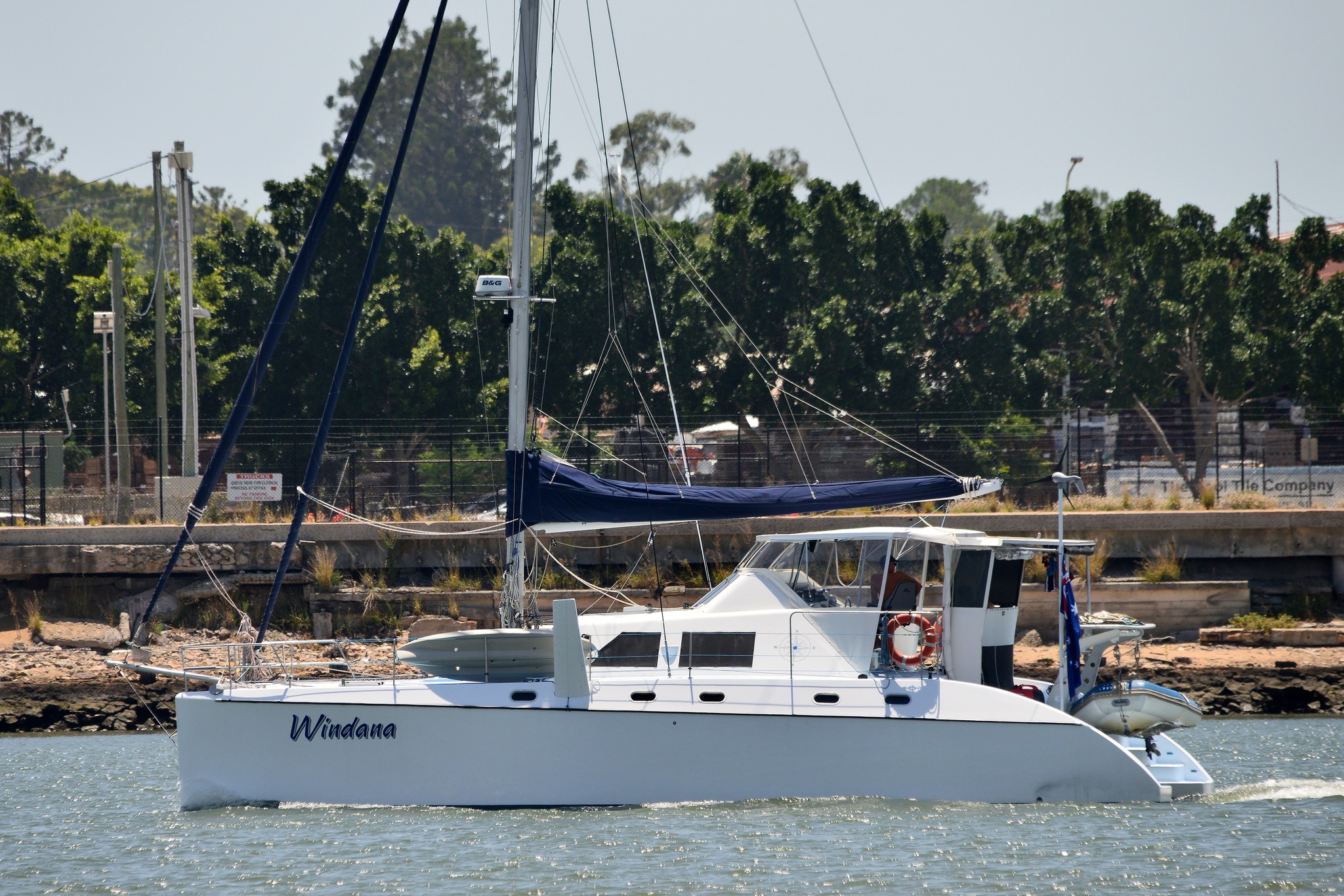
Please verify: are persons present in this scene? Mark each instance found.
[870,555,923,650]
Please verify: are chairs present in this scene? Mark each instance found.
[867,581,918,628]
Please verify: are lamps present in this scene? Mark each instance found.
[996,547,1034,561]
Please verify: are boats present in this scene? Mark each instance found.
[1064,675,1202,736]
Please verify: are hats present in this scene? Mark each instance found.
[879,554,899,566]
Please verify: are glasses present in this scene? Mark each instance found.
[889,562,897,566]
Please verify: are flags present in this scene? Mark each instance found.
[1060,559,1082,701]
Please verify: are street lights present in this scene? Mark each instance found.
[168,140,211,478]
[1066,156,1084,203]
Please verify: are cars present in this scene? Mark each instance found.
[464,488,506,514]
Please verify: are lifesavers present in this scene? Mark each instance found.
[933,616,943,654]
[886,613,936,666]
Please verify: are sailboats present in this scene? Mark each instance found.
[101,0,1218,812]
